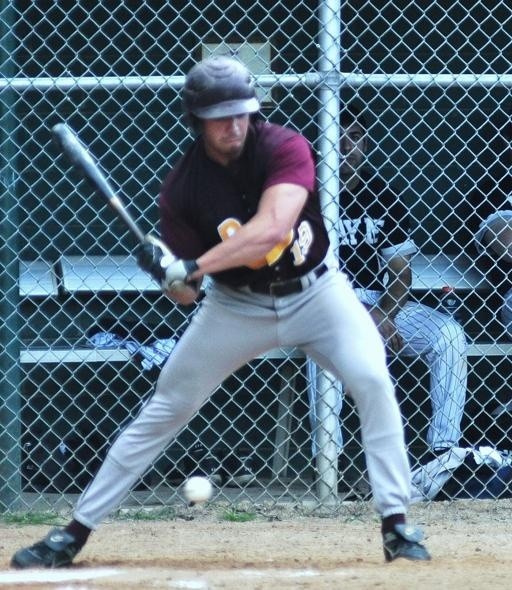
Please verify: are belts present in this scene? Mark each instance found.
[231,263,334,295]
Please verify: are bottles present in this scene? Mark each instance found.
[439,286,464,328]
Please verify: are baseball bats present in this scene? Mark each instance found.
[53,120,184,296]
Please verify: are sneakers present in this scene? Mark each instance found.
[9,527,76,569]
[378,524,432,565]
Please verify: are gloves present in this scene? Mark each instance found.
[163,257,197,288]
[136,235,173,275]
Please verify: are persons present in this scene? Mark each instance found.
[304,104,472,495]
[469,177,511,430]
[10,54,432,569]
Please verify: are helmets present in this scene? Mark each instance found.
[183,56,261,123]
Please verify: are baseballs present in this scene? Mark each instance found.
[183,476,212,500]
